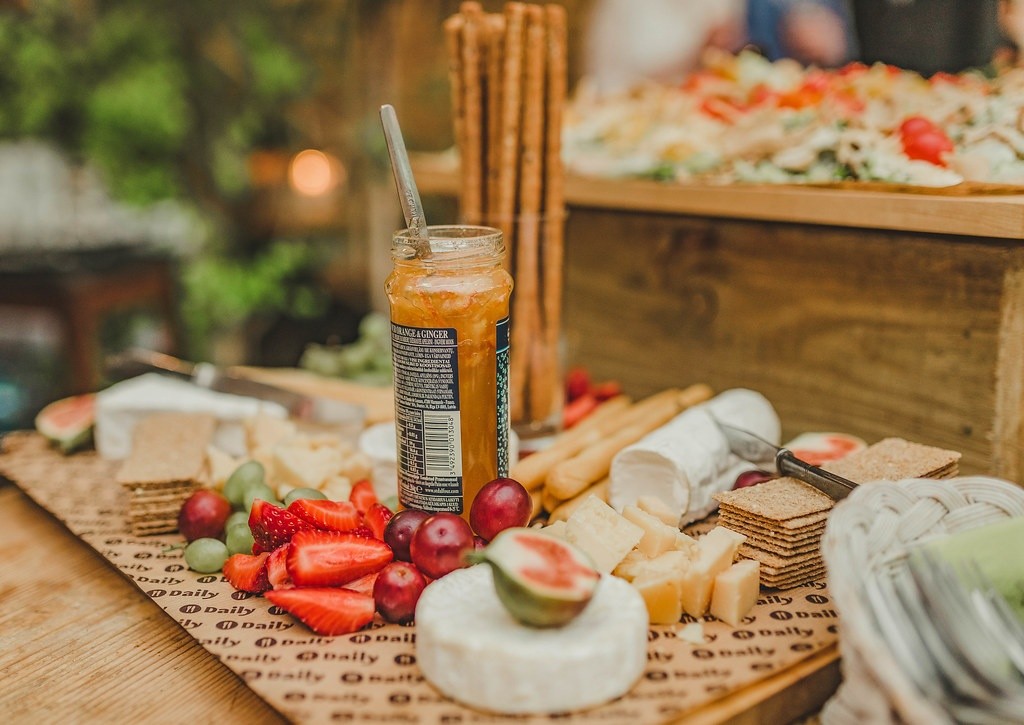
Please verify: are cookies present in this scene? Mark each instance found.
[709,437,963,590]
[118,414,216,533]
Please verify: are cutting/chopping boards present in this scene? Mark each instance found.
[667,644,845,725]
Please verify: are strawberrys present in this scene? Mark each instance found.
[224,478,395,635]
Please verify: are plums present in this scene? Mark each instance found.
[373,477,534,624]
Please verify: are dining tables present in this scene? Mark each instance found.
[0,366,953,725]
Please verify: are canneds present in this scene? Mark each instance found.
[384,225,514,514]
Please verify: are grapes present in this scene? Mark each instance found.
[183,460,328,576]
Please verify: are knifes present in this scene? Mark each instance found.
[718,420,860,501]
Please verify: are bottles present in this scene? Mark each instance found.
[384,225,514,544]
